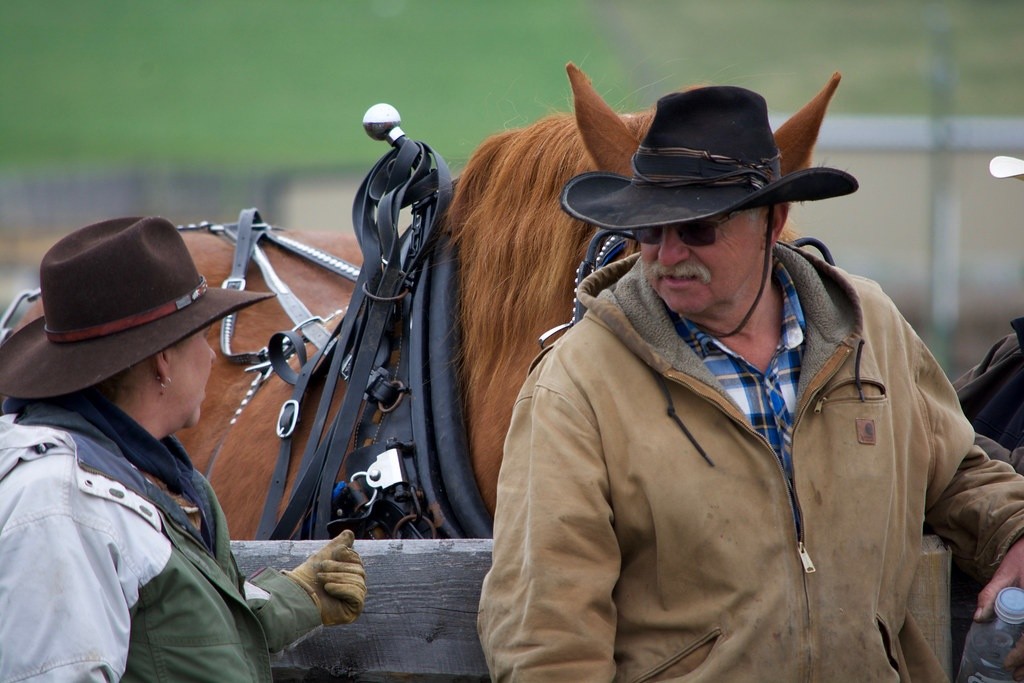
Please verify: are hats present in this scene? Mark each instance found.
[560,86,859,232]
[0,216,277,398]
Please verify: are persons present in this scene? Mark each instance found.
[0,215,368,683]
[477,84,1024,683]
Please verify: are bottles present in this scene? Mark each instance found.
[956,588,1024,683]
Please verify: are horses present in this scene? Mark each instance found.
[1,63,844,540]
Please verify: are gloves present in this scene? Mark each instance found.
[279,530,368,626]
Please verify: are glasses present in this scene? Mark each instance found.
[632,210,745,246]
